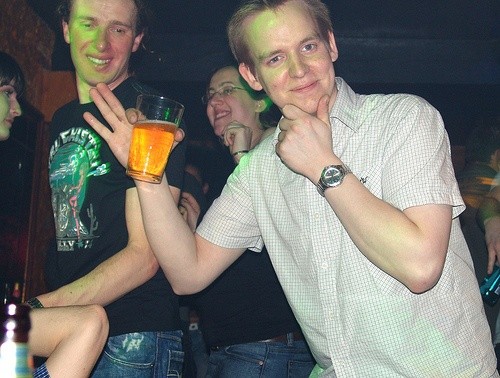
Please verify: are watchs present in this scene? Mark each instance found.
[317,164,352,197]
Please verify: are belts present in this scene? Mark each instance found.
[256,332,305,351]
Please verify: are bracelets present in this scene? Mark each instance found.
[233,150,249,156]
[26,297,44,308]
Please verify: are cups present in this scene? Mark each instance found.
[126,94,185,184]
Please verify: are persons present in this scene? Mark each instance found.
[25,0,187,378]
[179,63,317,378]
[457,125,500,337]
[83,0,500,378]
[0,51,23,138]
[26,303,110,378]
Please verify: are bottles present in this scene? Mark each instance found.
[479,265,500,308]
[0,283,34,378]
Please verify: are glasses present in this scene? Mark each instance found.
[200,86,252,105]
